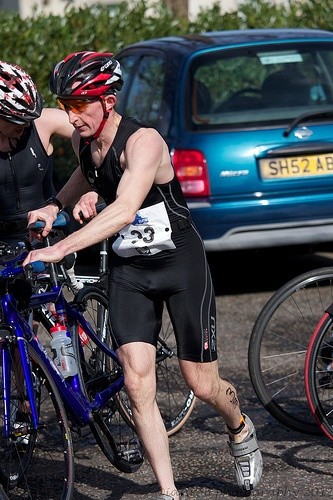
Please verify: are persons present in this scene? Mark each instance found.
[0,62,82,461]
[23,51,263,500]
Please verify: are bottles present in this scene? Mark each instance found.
[77,326,89,348]
[50,323,78,377]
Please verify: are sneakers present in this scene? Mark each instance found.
[226,413,264,492]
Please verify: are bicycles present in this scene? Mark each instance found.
[0,208,199,500]
[247,266,333,443]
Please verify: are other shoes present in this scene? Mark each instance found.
[7,421,37,448]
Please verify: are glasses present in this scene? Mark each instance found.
[54,96,100,115]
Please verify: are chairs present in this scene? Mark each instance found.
[260,71,311,109]
[194,80,212,116]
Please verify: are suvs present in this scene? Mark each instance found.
[112,25,333,272]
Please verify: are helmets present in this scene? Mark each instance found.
[48,50,125,98]
[0,60,43,124]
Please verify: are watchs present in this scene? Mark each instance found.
[46,196,63,213]
